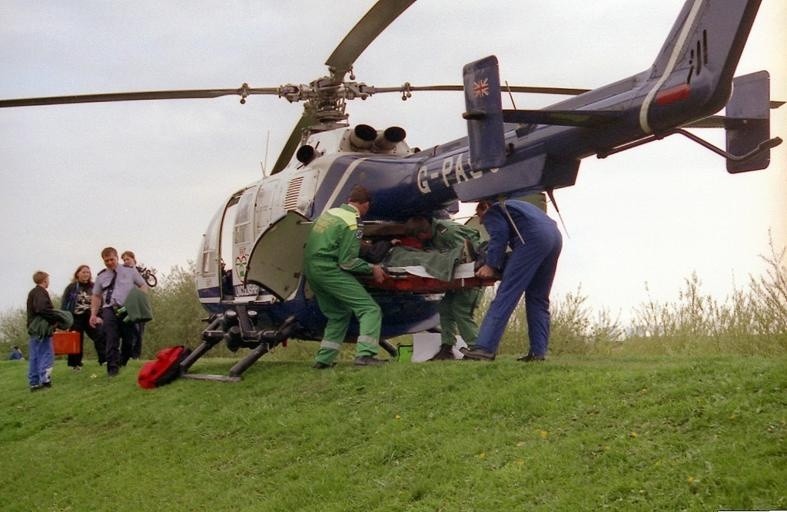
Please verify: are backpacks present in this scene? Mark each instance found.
[138,344,193,389]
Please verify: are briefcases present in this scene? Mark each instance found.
[52,330,83,355]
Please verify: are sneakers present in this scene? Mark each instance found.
[426,351,454,362]
[313,360,338,369]
[516,355,545,362]
[354,355,389,366]
[460,345,495,360]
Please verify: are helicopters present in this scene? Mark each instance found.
[1,0,787,386]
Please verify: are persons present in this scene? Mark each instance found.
[358,238,477,266]
[403,214,488,362]
[9,345,23,360]
[26,271,55,392]
[89,247,148,380]
[458,199,563,363]
[302,183,391,370]
[59,264,108,371]
[121,250,149,362]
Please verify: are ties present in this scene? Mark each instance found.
[101,270,117,304]
